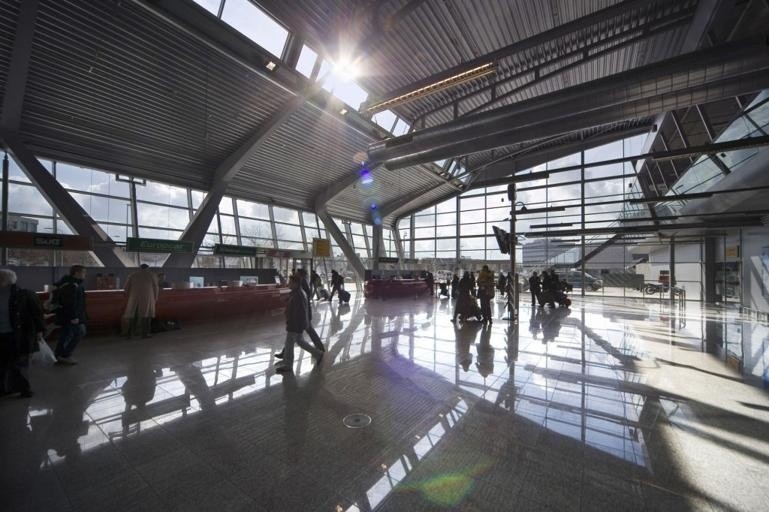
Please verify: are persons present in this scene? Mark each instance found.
[0,268,43,399]
[54,265,87,365]
[96,263,169,339]
[421,265,571,325]
[274,269,344,373]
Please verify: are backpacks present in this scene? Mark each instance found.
[39,283,64,315]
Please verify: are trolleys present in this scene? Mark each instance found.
[436,279,451,299]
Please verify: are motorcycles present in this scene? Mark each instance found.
[645,283,668,295]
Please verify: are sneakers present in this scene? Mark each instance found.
[450,317,493,325]
[7,388,38,403]
[126,332,139,341]
[311,343,328,375]
[274,346,292,360]
[54,355,80,368]
[530,304,556,311]
[274,364,294,375]
[141,331,155,342]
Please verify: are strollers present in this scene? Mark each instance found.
[458,289,483,324]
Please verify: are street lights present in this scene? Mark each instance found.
[403,234,407,253]
[43,226,62,234]
[123,203,133,242]
[503,201,528,320]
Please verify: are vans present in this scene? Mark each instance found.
[437,269,450,279]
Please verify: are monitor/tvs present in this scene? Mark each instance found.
[491,225,510,253]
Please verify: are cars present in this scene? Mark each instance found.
[560,269,604,291]
